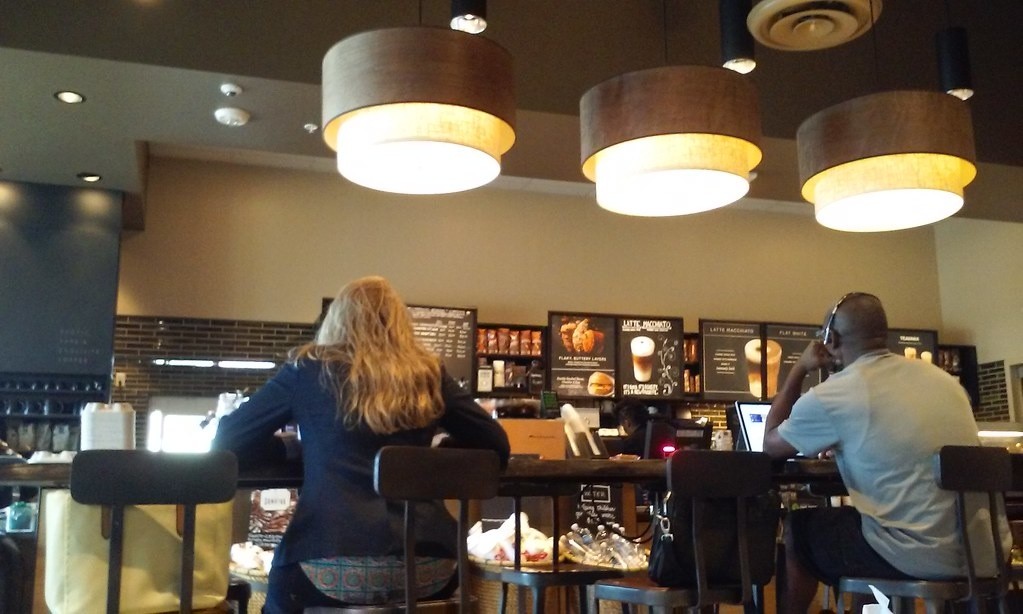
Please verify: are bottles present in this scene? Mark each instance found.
[560,521,646,567]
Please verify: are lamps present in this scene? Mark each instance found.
[578,0,764,218]
[935,0,975,101]
[449,0,488,33]
[718,0,756,74]
[794,0,977,233]
[321,0,517,193]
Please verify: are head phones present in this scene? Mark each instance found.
[824,292,879,345]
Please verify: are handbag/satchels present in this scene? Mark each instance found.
[646,491,696,588]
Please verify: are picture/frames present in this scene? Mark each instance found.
[887,329,938,368]
[404,304,477,397]
[617,313,685,401]
[547,309,617,401]
[764,321,828,404]
[698,317,764,405]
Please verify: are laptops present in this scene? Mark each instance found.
[734,400,807,459]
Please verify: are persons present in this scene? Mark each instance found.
[610,395,656,506]
[762,291,1014,614]
[210,276,512,614]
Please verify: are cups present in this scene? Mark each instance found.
[744,339,782,397]
[1,380,103,391]
[630,336,654,383]
[905,346,917,359]
[921,351,932,363]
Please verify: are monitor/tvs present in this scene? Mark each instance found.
[645,417,712,460]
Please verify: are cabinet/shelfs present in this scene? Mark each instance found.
[475,323,547,397]
[681,331,700,403]
[935,342,979,413]
[0,372,114,459]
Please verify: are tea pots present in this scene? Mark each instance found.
[1,396,93,415]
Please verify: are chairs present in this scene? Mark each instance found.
[0,446,1023,614]
